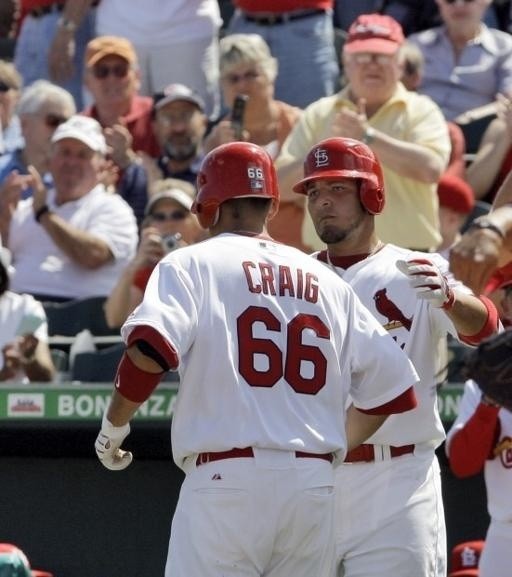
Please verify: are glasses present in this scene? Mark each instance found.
[0,83,10,91]
[151,210,187,221]
[357,54,391,65]
[94,65,127,78]
[46,115,66,127]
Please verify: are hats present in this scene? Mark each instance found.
[0,543,52,576]
[84,36,135,68]
[145,178,196,211]
[151,83,206,113]
[449,541,485,577]
[437,175,474,214]
[52,115,106,155]
[344,15,405,55]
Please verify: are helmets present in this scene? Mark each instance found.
[292,137,384,213]
[192,141,280,229]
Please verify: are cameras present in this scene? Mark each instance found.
[160,234,181,253]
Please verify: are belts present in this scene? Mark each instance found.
[196,446,334,463]
[344,445,414,462]
[244,8,325,26]
[31,3,65,18]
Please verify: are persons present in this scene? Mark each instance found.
[288,134,510,574]
[1,0,511,397]
[92,135,420,574]
[442,265,511,576]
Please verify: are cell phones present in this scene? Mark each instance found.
[229,94,245,138]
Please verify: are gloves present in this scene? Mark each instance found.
[94,410,133,470]
[397,258,454,310]
